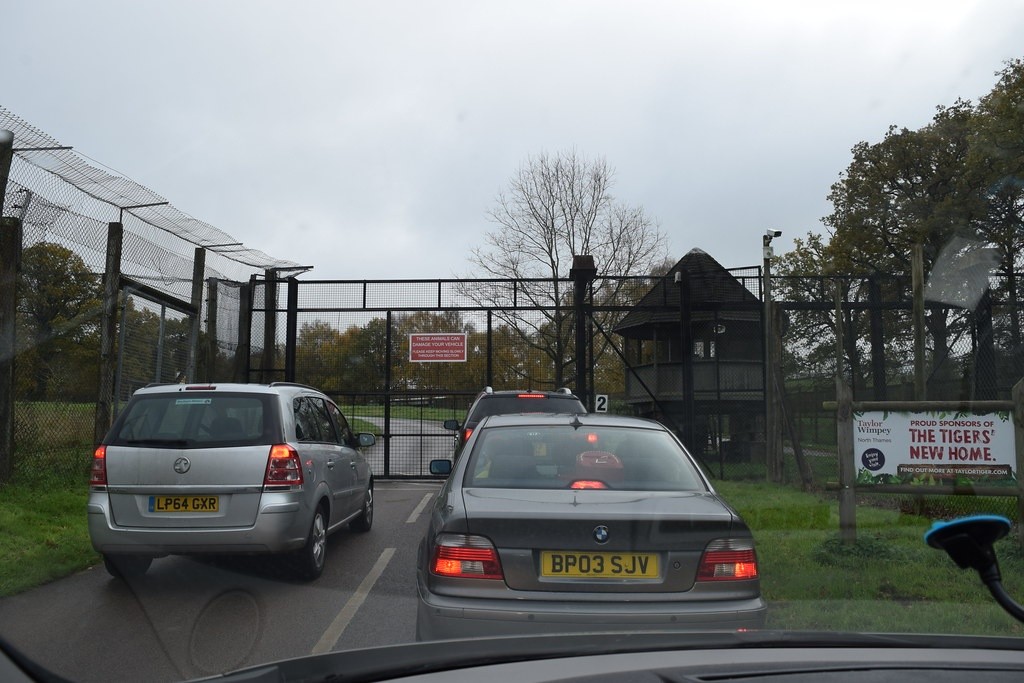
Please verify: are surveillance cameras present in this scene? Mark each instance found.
[767,229,782,237]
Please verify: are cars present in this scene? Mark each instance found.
[87,379,376,583]
[416,412,767,643]
[444,386,587,463]
